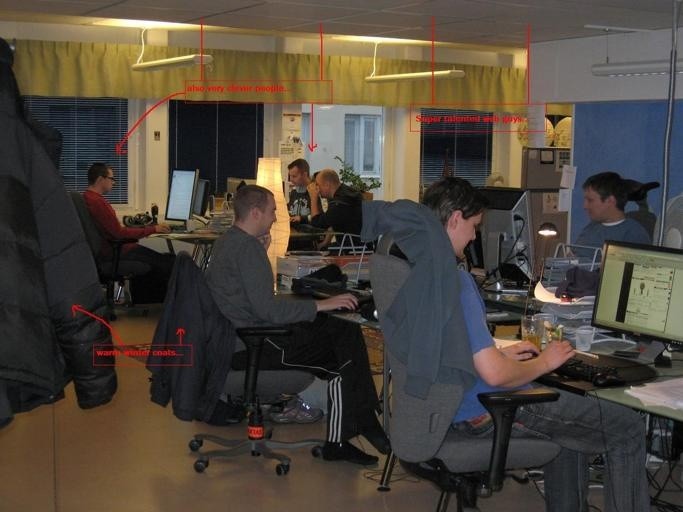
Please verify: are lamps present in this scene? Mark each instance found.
[365,43,465,84]
[591,26,683,78]
[132,29,212,72]
[537,224,559,238]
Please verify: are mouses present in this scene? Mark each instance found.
[338,301,355,315]
[593,371,626,388]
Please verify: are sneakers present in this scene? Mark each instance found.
[268,395,324,424]
[363,424,392,455]
[322,440,379,465]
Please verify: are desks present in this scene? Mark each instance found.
[150,213,328,256]
[281,274,683,422]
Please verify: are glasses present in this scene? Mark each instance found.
[102,175,115,182]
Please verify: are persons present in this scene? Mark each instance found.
[305,171,362,253]
[84,162,175,285]
[200,185,388,464]
[287,159,318,224]
[420,179,651,511]
[565,173,650,263]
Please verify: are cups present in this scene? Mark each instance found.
[151,205,158,224]
[521,313,595,353]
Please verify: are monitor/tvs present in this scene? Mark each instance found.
[464,187,537,292]
[591,238,683,366]
[165,166,199,224]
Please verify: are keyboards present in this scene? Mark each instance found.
[554,357,621,382]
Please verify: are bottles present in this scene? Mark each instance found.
[295,266,304,279]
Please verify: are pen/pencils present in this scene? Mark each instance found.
[573,349,599,360]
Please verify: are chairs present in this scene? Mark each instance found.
[325,232,370,255]
[367,252,563,510]
[67,189,149,321]
[627,211,659,242]
[176,249,329,476]
